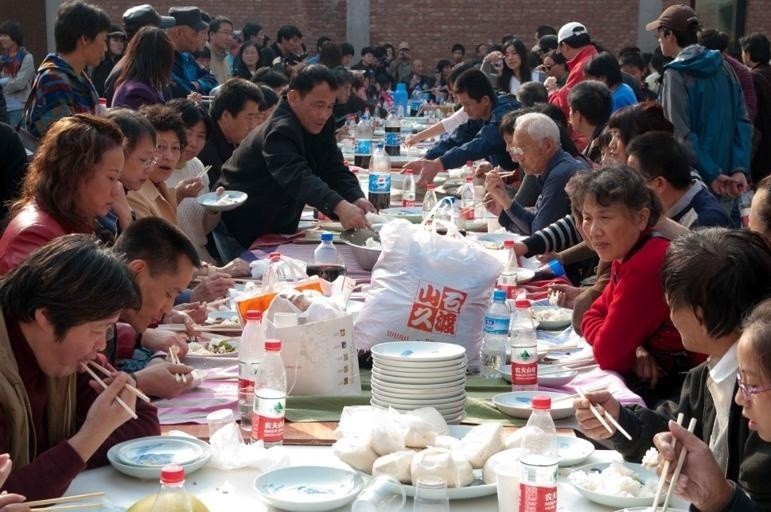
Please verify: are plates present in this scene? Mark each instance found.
[173,309,245,358]
[476,233,581,420]
[332,424,500,501]
[295,206,348,233]
[513,435,592,465]
[569,459,677,506]
[253,463,362,510]
[105,435,212,478]
[196,188,249,212]
[371,341,470,425]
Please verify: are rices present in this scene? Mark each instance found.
[569,446,666,498]
[362,237,381,250]
[535,295,569,321]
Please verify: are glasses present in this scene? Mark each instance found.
[510,142,535,156]
[736,370,771,400]
[143,157,159,169]
[542,63,557,71]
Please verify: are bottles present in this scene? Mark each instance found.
[97,97,110,116]
[312,232,348,279]
[236,310,290,449]
[148,463,200,510]
[518,393,559,510]
[262,252,295,284]
[347,85,476,227]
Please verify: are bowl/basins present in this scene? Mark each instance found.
[340,223,383,271]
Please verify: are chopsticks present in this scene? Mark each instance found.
[167,343,188,387]
[187,94,216,103]
[461,196,495,216]
[76,358,151,421]
[9,490,111,510]
[485,171,515,180]
[577,389,633,441]
[192,164,212,184]
[539,341,609,401]
[648,412,698,510]
[155,272,264,333]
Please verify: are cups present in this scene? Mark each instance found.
[206,409,239,466]
[349,477,452,509]
[494,460,520,510]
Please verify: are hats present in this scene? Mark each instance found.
[169,7,209,30]
[646,5,699,32]
[557,22,588,54]
[201,9,217,27]
[398,41,410,51]
[107,25,127,38]
[122,4,176,31]
[532,35,558,52]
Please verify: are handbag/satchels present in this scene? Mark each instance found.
[256,311,361,398]
[14,125,42,163]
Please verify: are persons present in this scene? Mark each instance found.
[1,1,771,512]
